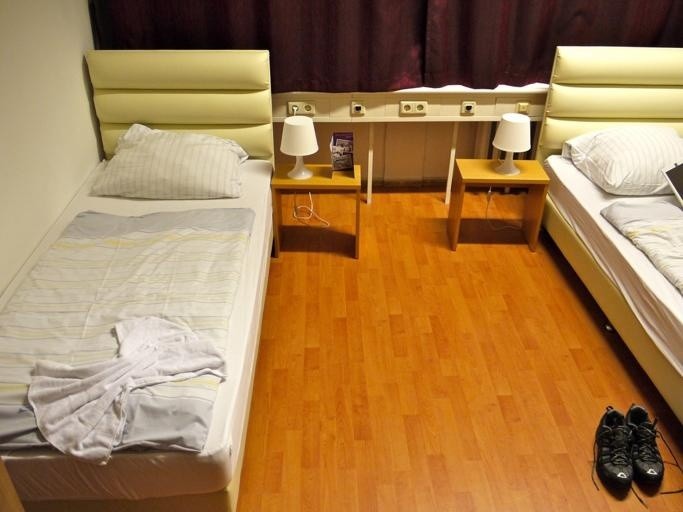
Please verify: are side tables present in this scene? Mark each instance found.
[271,164,362,258]
[448,158,550,251]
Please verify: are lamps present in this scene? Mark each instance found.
[492,113,532,176]
[280,115,319,180]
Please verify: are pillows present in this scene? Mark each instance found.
[562,127,683,196]
[91,123,248,200]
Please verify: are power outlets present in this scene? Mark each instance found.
[399,100,428,114]
[288,102,315,115]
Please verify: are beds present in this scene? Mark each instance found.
[535,45,683,425]
[0,49,274,512]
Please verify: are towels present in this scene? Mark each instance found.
[26,317,227,465]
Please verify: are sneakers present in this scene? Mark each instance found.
[591,403,664,490]
